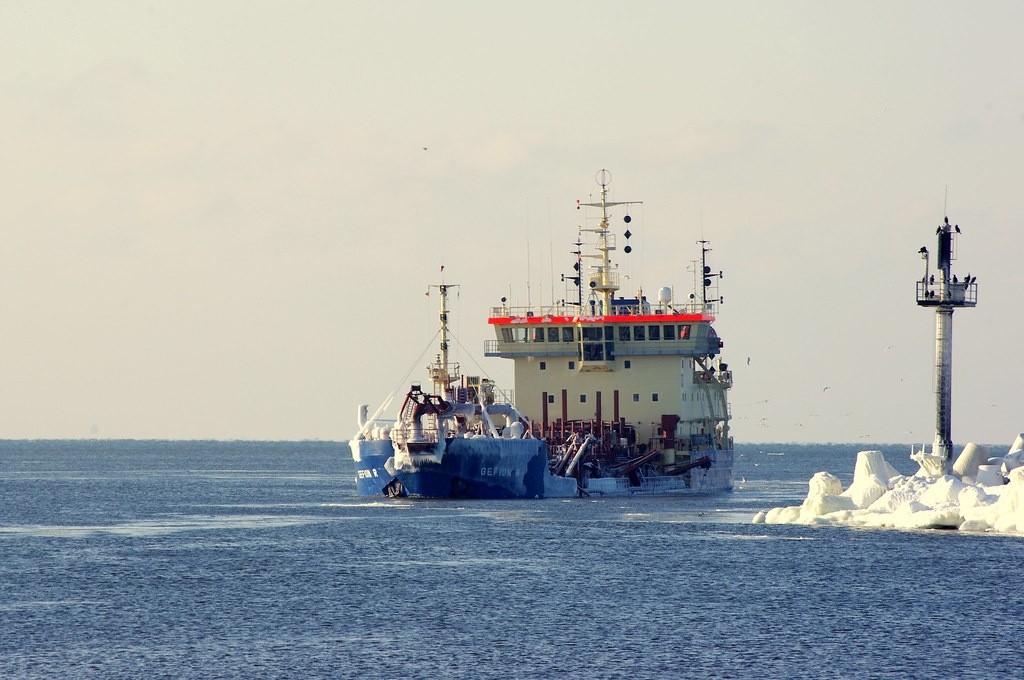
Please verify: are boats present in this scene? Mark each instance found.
[481,169,734,500]
[348,263,551,501]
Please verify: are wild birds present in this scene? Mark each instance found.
[918,246,928,254]
[952,273,976,290]
[936,215,963,235]
[741,475,746,484]
[822,386,831,393]
[746,356,751,367]
[922,274,935,299]
